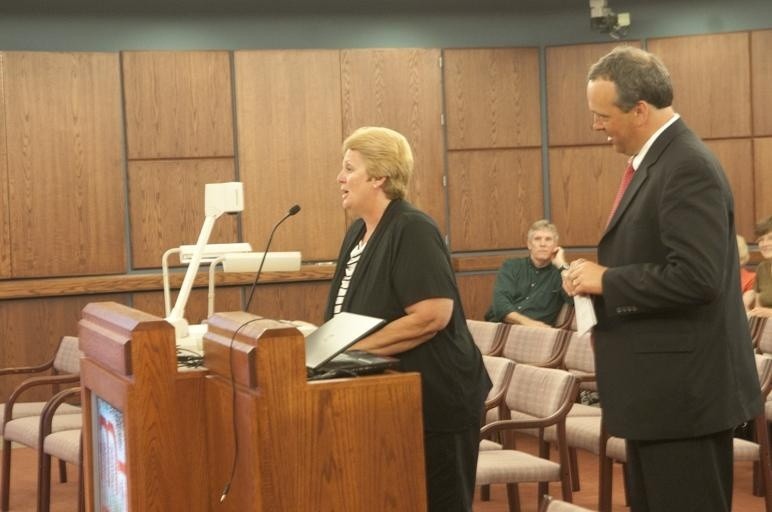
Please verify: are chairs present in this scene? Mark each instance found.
[1,302,772,512]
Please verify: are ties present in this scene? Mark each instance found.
[602,158,637,239]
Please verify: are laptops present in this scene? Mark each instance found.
[304,311,401,377]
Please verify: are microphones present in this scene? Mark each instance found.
[244,205,302,314]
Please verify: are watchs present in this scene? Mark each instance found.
[556,262,570,274]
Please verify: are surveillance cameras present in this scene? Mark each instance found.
[589,0,618,32]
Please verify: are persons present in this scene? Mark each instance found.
[323,126,494,512]
[735,233,755,311]
[559,43,764,511]
[743,216,771,320]
[483,216,574,329]
